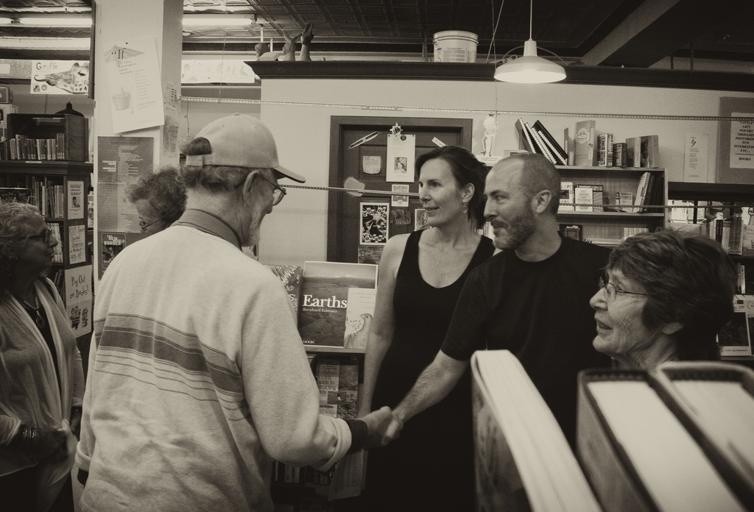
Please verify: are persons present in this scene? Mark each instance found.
[358,143,500,511]
[0,201,86,511]
[72,111,394,511]
[376,149,614,447]
[588,228,729,369]
[121,164,184,242]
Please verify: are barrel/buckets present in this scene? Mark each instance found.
[431,29,481,63]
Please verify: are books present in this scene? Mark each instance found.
[570,361,744,511]
[511,115,753,267]
[255,262,375,492]
[639,353,752,509]
[9,109,69,298]
[457,348,602,509]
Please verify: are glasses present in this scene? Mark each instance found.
[20,228,54,243]
[262,172,287,206]
[598,273,649,302]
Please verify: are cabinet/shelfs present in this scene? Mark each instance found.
[666,181,754,366]
[550,165,665,253]
[260,265,380,512]
[0,159,94,390]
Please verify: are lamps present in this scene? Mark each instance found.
[492,0,566,85]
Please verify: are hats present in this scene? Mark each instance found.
[181,114,308,183]
